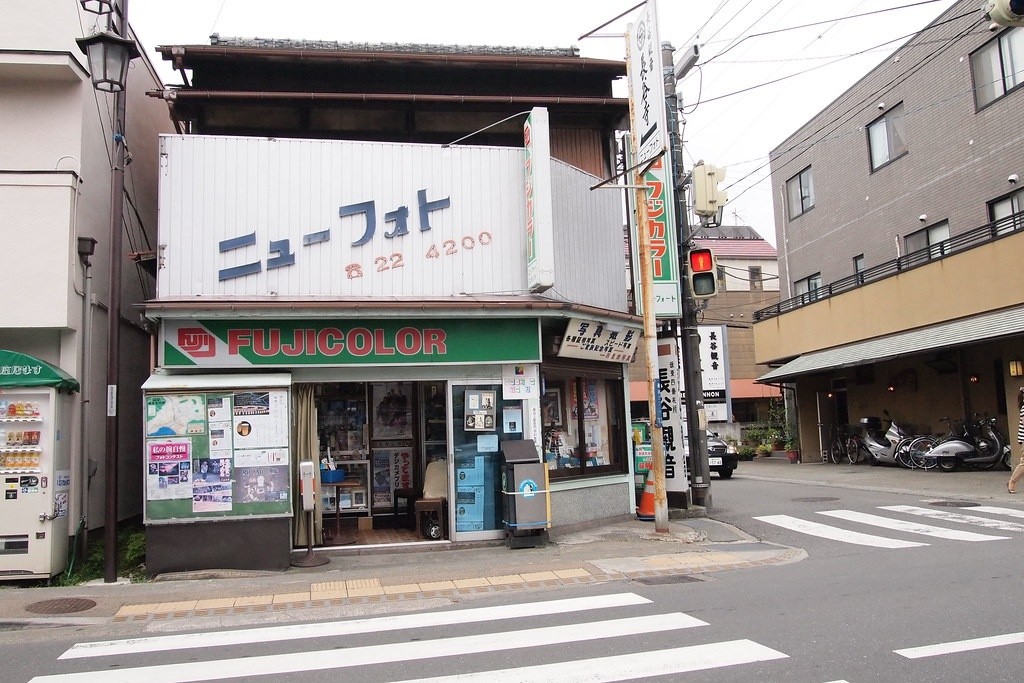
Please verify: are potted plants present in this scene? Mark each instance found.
[784,441,798,463]
[738,424,786,461]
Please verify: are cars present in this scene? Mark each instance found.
[680,417,740,479]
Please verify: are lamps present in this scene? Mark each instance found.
[1009,358,1023,377]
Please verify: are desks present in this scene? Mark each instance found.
[320,478,363,545]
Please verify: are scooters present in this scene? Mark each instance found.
[923,409,1011,472]
[858,410,922,469]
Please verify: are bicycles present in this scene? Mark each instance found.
[895,412,975,470]
[829,422,861,465]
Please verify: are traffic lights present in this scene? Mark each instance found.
[689,247,720,300]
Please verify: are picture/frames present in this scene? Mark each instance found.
[367,382,419,448]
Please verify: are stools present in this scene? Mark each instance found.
[415,498,446,540]
[393,487,421,531]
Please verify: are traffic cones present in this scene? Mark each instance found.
[636,462,656,521]
